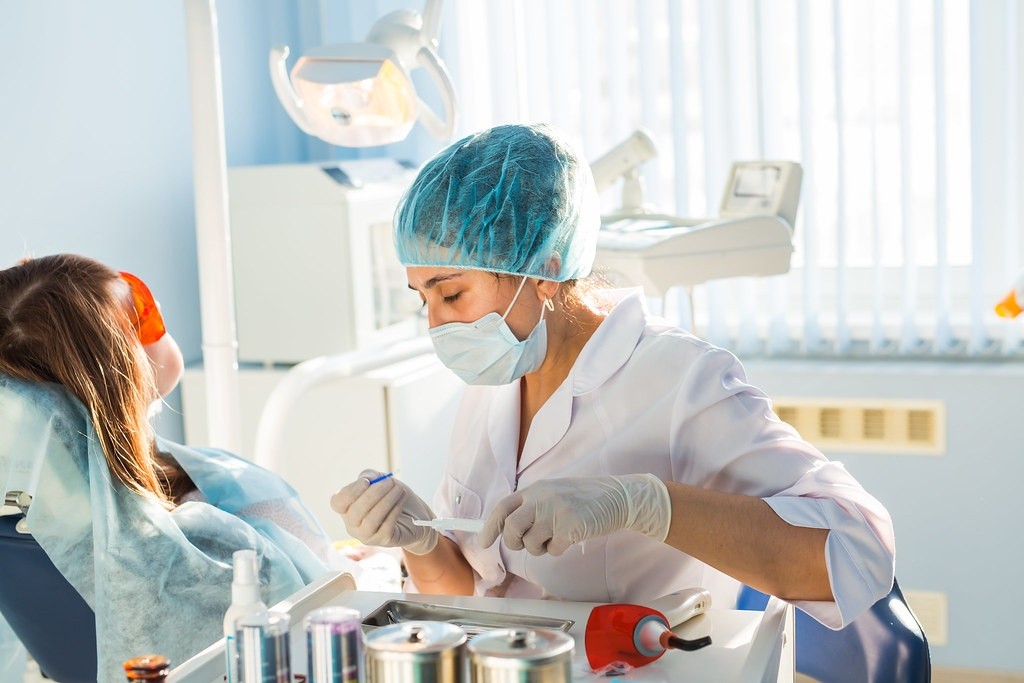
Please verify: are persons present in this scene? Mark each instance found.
[330,125,895,683]
[0,252,402,595]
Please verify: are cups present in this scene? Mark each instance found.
[362,619,468,683]
[468,625,577,683]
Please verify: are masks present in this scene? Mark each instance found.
[428,261,550,385]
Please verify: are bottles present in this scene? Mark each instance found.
[124,653,171,683]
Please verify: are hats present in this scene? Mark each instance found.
[392,123,600,281]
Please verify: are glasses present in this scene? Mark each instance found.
[122,271,167,349]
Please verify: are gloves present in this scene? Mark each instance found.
[477,472,672,554]
[331,470,440,553]
